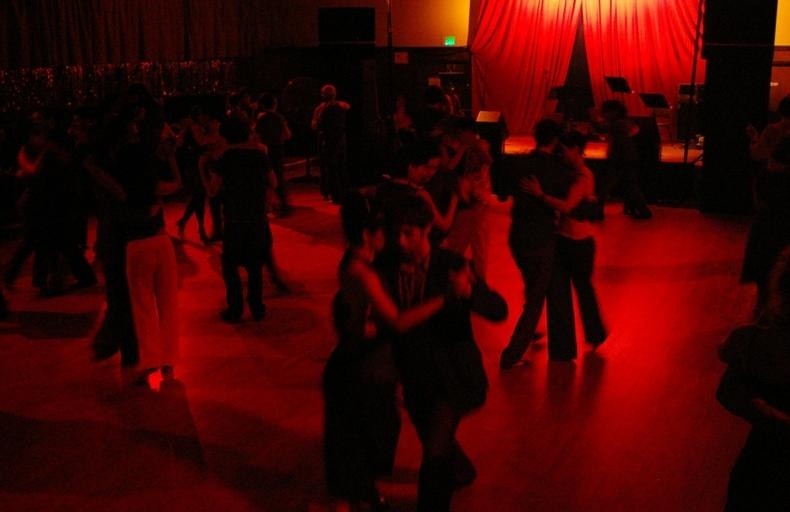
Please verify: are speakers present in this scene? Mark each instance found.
[319,7,375,42]
[477,110,509,139]
[700,48,772,216]
[704,0,777,47]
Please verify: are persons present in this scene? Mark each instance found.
[2,83,787,509]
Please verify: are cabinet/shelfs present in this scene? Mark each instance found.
[357,43,470,128]
